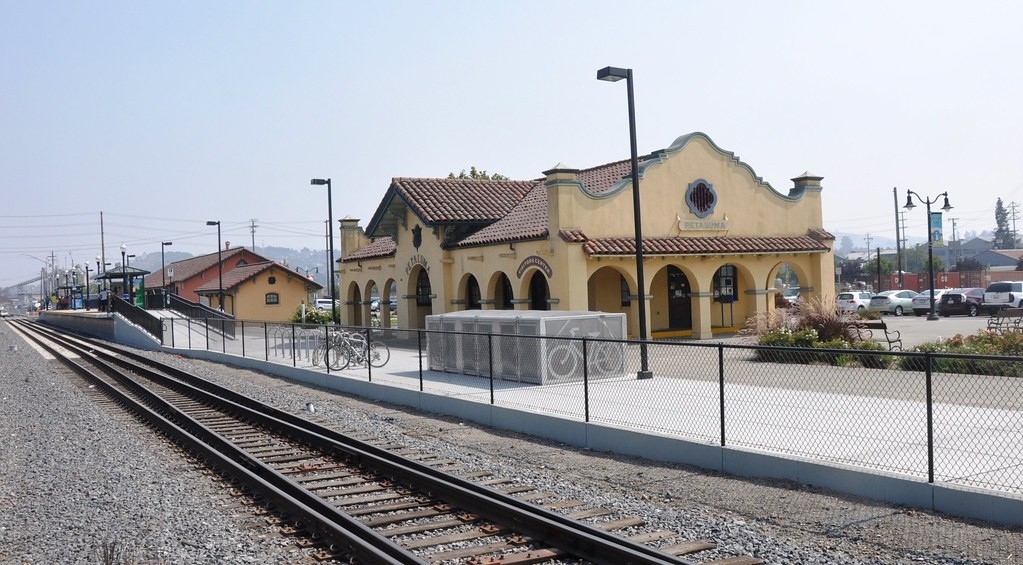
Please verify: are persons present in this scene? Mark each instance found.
[56,295,70,310]
[51,293,56,310]
[45,296,50,310]
[99,289,107,312]
[114,286,123,296]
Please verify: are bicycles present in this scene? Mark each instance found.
[312,326,390,372]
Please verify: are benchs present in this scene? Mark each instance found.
[845,322,903,352]
[986,304,1023,336]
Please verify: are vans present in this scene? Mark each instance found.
[981,281,1023,310]
[313,299,333,310]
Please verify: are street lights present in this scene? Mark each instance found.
[55,261,90,311]
[597,66,653,379]
[96,254,101,311]
[309,178,337,325]
[902,189,954,482]
[120,241,127,294]
[126,255,135,267]
[206,221,223,311]
[161,241,173,289]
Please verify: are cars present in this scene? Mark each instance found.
[782,287,802,304]
[836,286,986,317]
[390,300,397,311]
